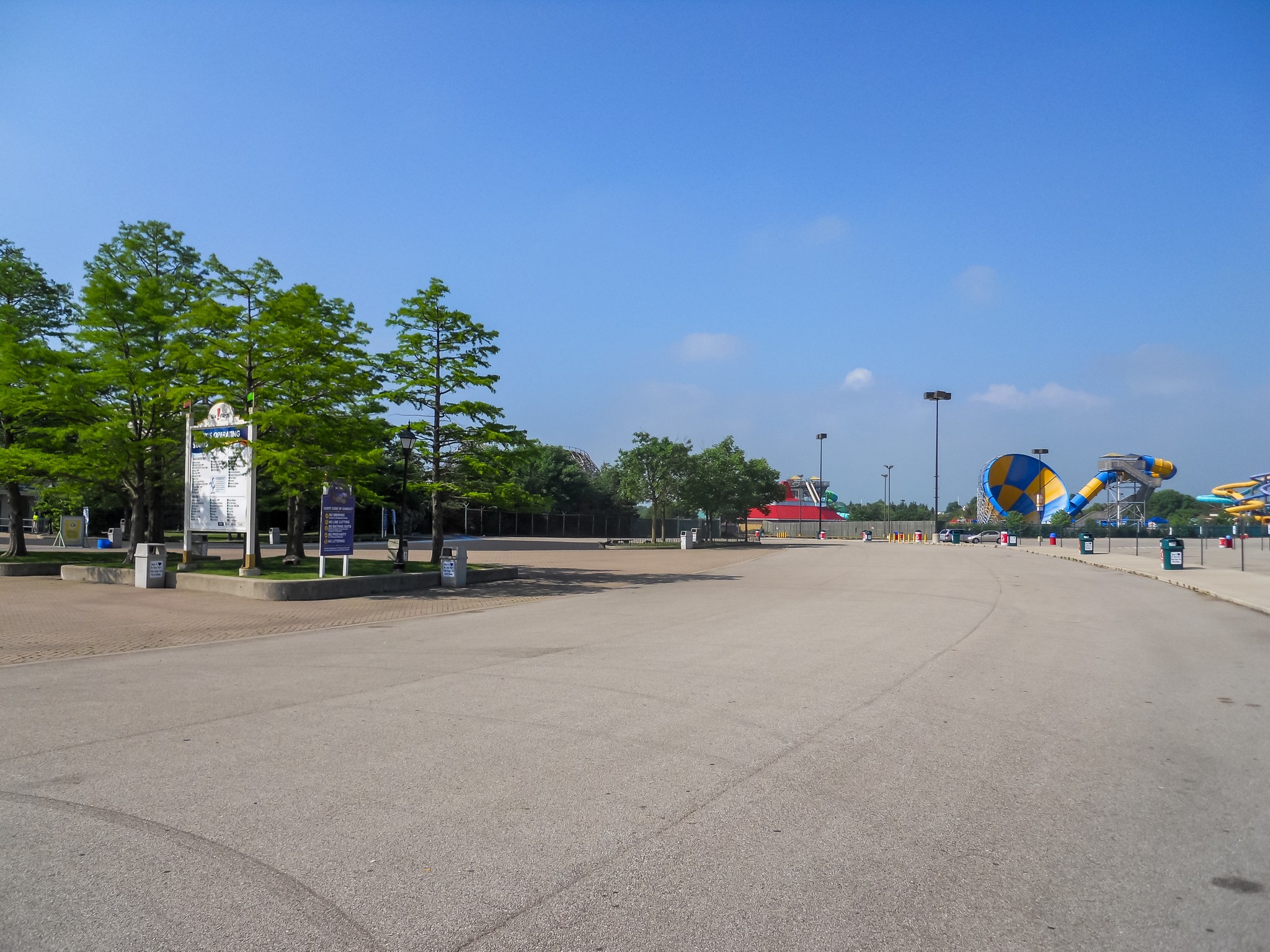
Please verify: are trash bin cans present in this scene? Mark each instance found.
[191,534,209,556]
[1079,531,1094,554]
[680,530,693,549]
[915,529,922,542]
[134,543,168,588]
[268,528,280,545]
[899,532,903,540]
[755,530,764,538]
[107,528,122,548]
[387,539,409,561]
[817,529,826,540]
[894,531,898,540]
[1001,530,1018,546]
[1159,536,1185,570]
[951,531,961,544]
[439,546,468,587]
[1219,535,1232,549]
[862,530,872,541]
[690,528,702,543]
[1049,533,1056,545]
[120,519,131,541]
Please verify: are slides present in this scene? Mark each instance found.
[1196,472,1270,526]
[822,491,838,504]
[977,453,1176,525]
[836,512,850,518]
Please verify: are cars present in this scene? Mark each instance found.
[967,530,1000,544]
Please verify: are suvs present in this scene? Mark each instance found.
[939,528,977,543]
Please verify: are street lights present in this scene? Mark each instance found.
[393,420,418,572]
[797,475,804,536]
[924,390,952,543]
[46,483,55,535]
[884,464,893,539]
[1031,449,1049,547]
[816,433,827,533]
[881,474,887,537]
